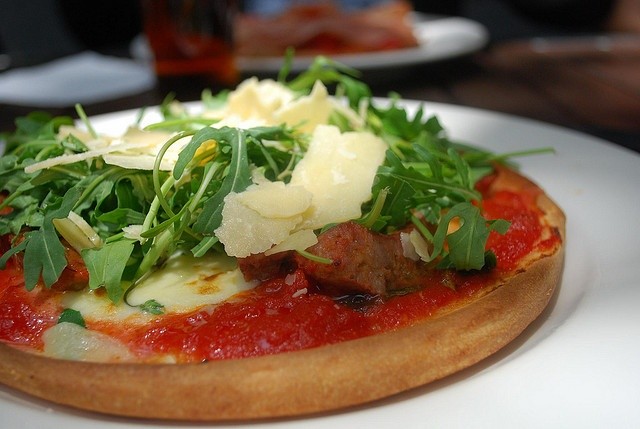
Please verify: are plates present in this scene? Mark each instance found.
[1,52,156,110]
[0,95,640,429]
[128,2,489,75]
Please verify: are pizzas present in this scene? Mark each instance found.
[0,47,567,421]
